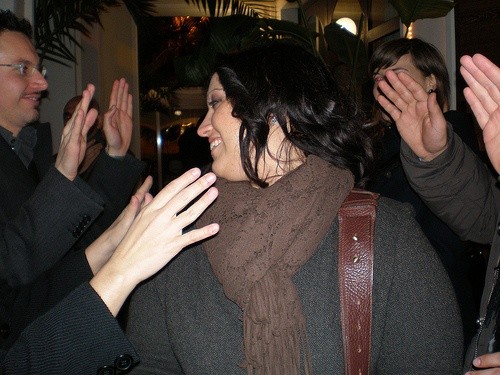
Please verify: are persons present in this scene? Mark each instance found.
[377,53,500,375]
[367,38,480,352]
[465,352,500,375]
[126,44,464,375]
[0,10,146,296]
[0,167,220,375]
[52,95,214,183]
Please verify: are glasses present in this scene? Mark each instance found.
[0,60,47,78]
